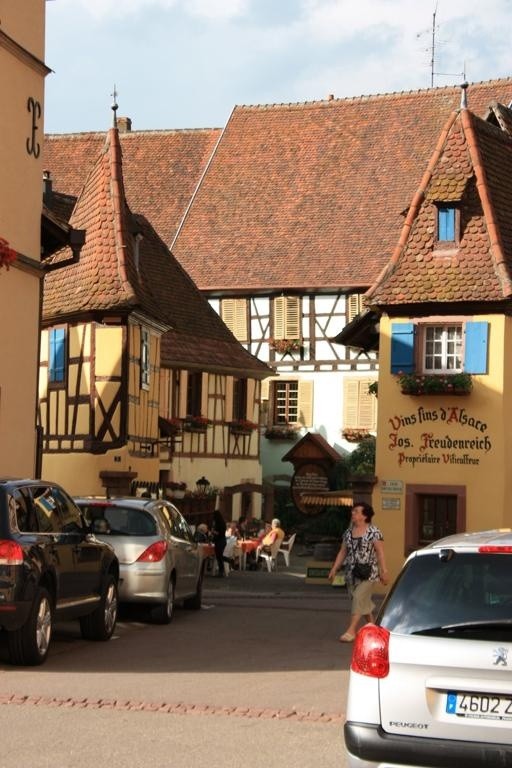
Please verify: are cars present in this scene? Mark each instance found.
[344,529,512,765]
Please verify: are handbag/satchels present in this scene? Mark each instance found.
[351,563,371,580]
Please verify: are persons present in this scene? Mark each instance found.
[326,502,392,643]
[209,508,235,576]
[187,517,285,569]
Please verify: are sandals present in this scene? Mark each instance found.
[340,633,357,642]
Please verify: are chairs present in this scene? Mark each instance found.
[203,533,296,575]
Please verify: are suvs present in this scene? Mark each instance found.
[73,496,207,624]
[0,478,119,666]
